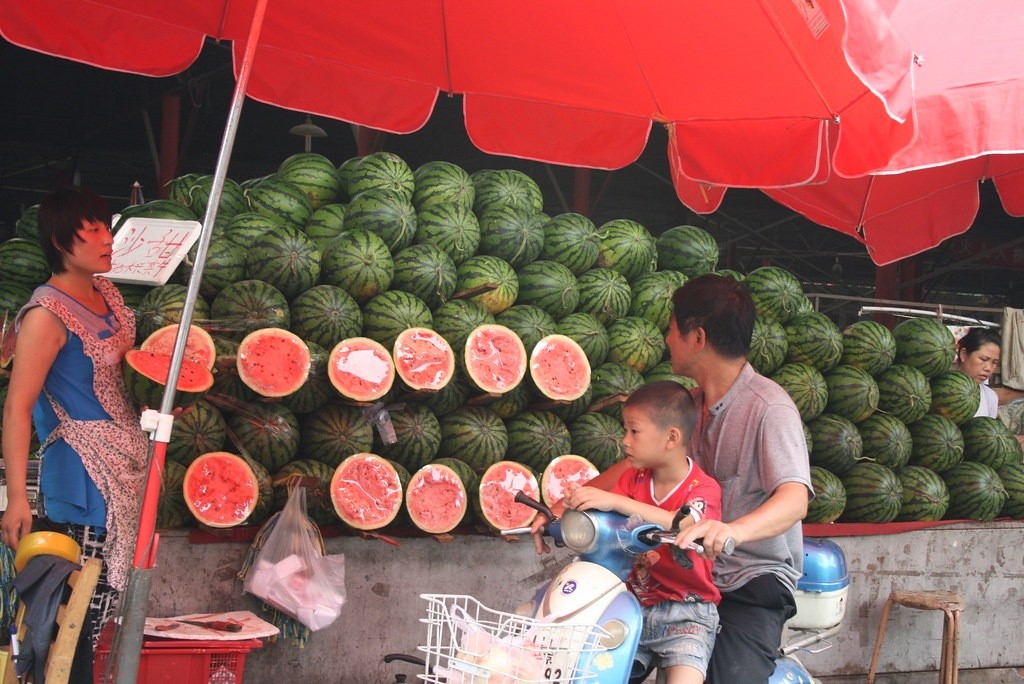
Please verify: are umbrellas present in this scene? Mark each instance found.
[0,1,1023,684]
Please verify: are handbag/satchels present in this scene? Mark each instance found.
[242,472,350,636]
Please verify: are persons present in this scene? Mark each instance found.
[1,184,151,682]
[530,276,816,684]
[564,378,724,684]
[950,326,1004,422]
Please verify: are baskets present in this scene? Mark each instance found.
[416,592,613,684]
[94,644,252,684]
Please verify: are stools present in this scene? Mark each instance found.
[863,588,967,684]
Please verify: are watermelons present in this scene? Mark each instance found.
[1,152,1024,536]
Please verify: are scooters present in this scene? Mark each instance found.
[418,490,852,684]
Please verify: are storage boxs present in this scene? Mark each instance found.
[92,617,265,684]
